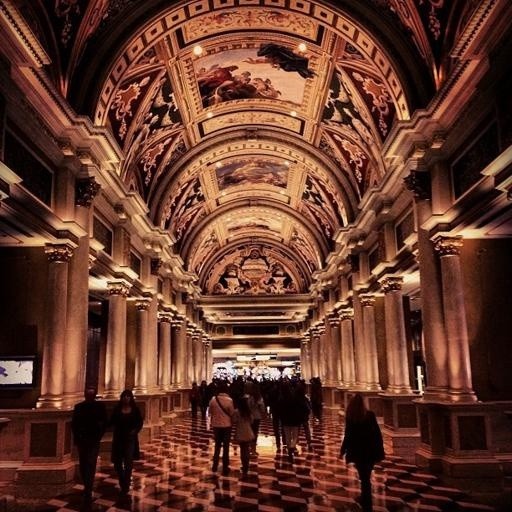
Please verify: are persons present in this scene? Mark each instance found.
[110,390,144,506]
[71,385,107,504]
[188,363,323,475]
[339,393,385,512]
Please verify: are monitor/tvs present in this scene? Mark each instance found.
[0,357,35,388]
